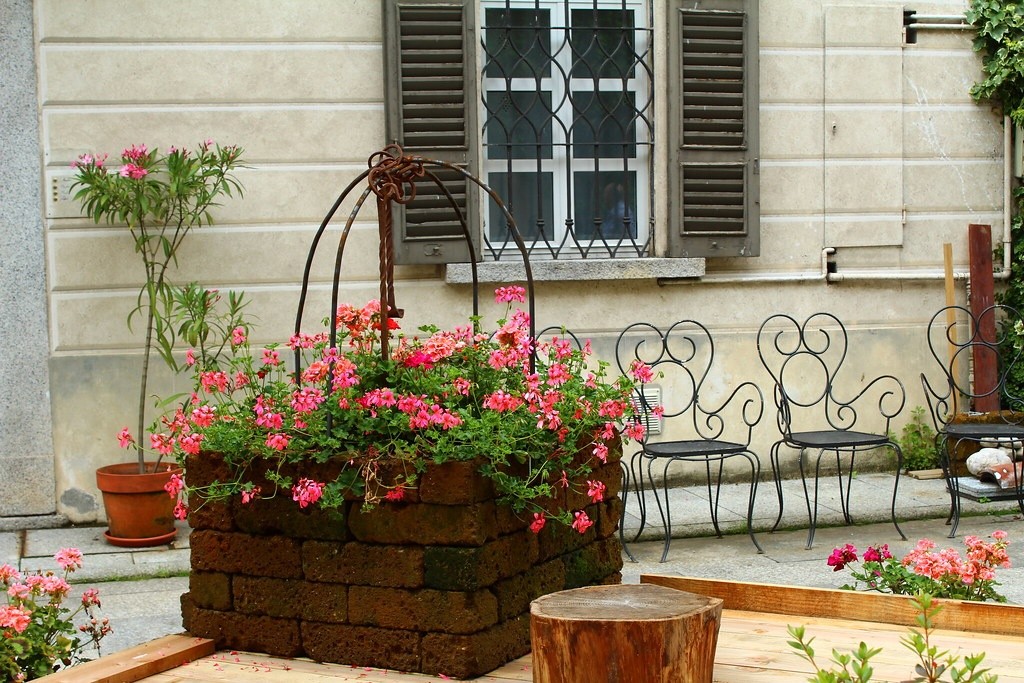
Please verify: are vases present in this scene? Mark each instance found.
[96,463,184,545]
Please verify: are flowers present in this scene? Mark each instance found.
[67,137,258,473]
[111,285,664,532]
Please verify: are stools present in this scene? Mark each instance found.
[531,583,725,683]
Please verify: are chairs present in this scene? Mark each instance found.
[920,306,1024,538]
[757,312,908,550]
[534,318,764,562]
